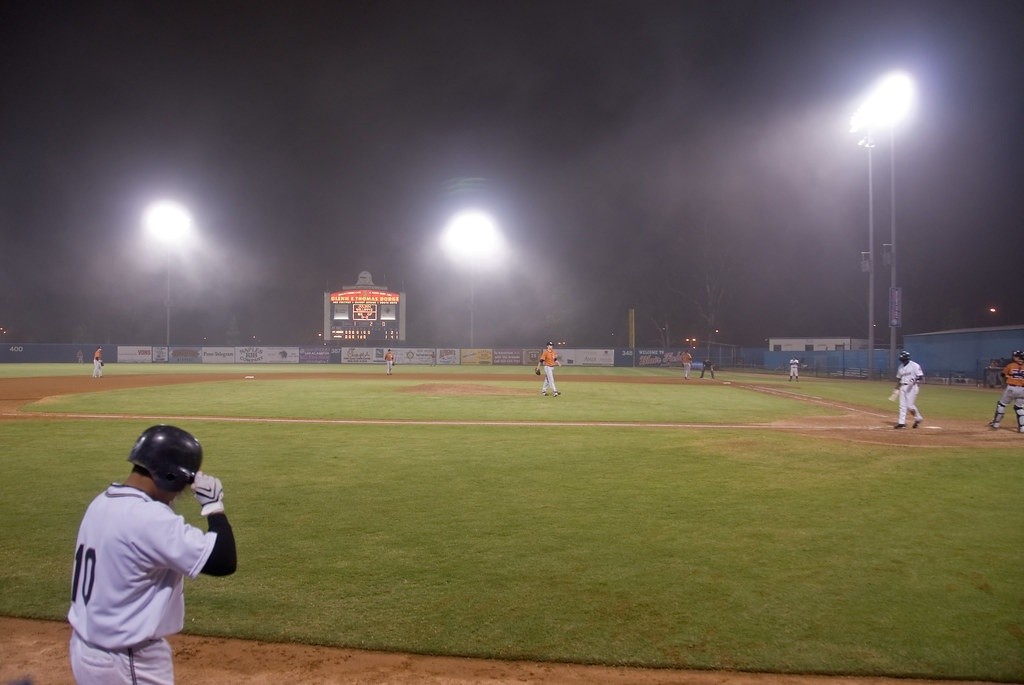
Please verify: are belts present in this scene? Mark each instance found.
[901,383,908,385]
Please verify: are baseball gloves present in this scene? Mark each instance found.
[682,363,684,367]
[101,362,104,366]
[535,367,541,375]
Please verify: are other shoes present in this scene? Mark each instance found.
[553,391,561,396]
[894,424,906,429]
[542,392,549,396]
[912,420,921,428]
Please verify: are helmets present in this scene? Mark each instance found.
[899,351,910,361]
[127,425,202,492]
[1012,350,1024,359]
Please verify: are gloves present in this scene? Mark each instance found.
[190,471,224,516]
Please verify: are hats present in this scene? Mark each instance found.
[547,341,553,345]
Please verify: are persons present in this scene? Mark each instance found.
[92,345,103,378]
[77,349,84,363]
[682,350,692,380]
[431,352,436,367]
[700,360,714,379]
[68,424,237,685]
[893,351,924,429]
[789,355,799,383]
[535,341,561,397]
[385,349,394,375]
[990,350,1024,434]
